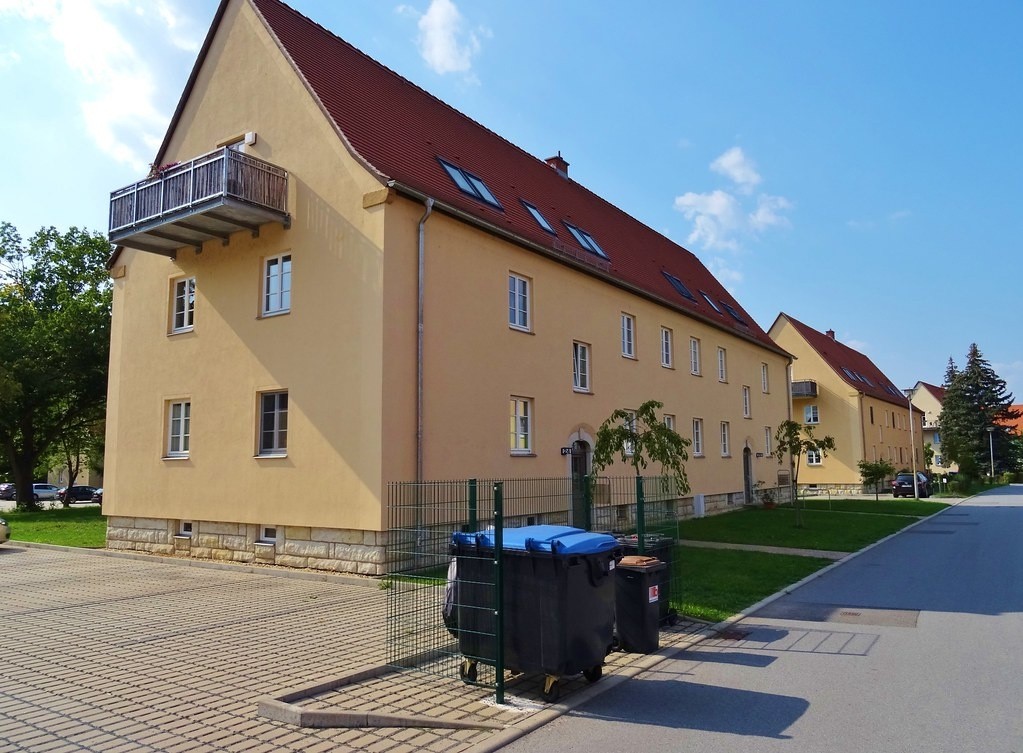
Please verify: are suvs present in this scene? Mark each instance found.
[891,472,933,498]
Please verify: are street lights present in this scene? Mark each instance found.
[901,388,919,499]
[986,427,995,477]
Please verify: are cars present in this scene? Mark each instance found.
[54,485,98,504]
[91,488,102,505]
[0,483,16,501]
[0,518,11,544]
[32,484,60,501]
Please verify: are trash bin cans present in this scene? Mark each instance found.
[449,521,625,702]
[617,553,667,656]
[617,530,679,628]
